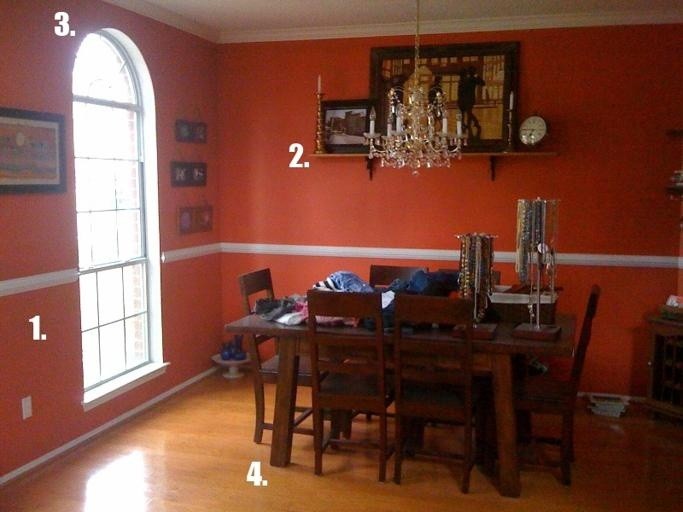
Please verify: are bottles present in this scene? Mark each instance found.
[222,333,246,360]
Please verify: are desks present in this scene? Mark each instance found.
[215,312,577,496]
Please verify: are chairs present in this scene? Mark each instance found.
[516,284,601,487]
[368,263,427,291]
[306,286,394,482]
[389,287,487,490]
[237,265,352,450]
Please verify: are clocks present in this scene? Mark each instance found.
[517,109,548,152]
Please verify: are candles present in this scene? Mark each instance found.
[317,72,321,92]
[508,88,513,109]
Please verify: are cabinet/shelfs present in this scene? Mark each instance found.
[645,314,682,429]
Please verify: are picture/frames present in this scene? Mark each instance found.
[173,204,214,237]
[170,157,206,189]
[0,108,67,194]
[321,100,378,154]
[365,42,522,152]
[173,118,207,147]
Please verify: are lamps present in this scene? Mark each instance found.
[363,4,465,170]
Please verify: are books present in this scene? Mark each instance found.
[587,395,630,418]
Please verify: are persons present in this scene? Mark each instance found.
[429,65,485,129]
